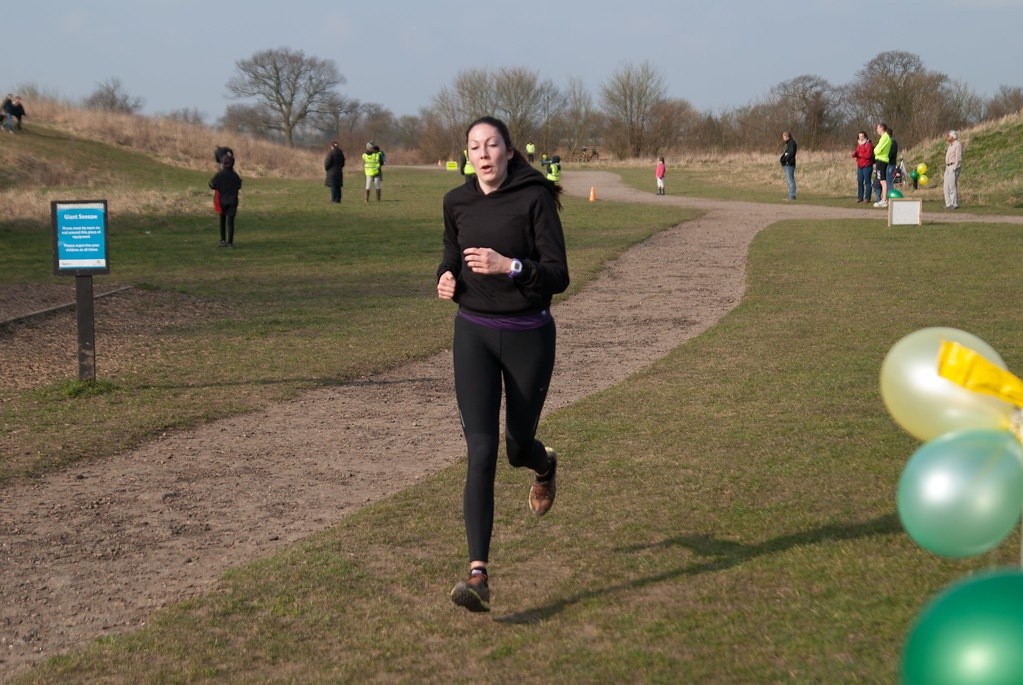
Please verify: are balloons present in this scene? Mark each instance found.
[897,565,1023,685]
[879,326,1023,561]
[912,163,928,185]
[887,189,904,198]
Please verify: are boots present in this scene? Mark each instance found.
[365,189,370,202]
[657,188,665,196]
[376,189,381,202]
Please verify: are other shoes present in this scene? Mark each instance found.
[856,198,862,203]
[217,240,225,246]
[950,205,958,209]
[784,197,796,201]
[863,199,870,204]
[225,243,232,247]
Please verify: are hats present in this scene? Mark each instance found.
[331,140,337,148]
[366,141,374,151]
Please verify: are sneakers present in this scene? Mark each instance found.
[449,574,490,612]
[874,201,887,207]
[529,447,557,515]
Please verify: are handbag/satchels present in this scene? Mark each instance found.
[214,190,220,212]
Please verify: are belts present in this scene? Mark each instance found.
[948,163,955,166]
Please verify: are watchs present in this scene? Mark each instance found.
[508,258,522,278]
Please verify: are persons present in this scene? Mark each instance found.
[459,149,477,182]
[582,146,599,162]
[208,143,242,247]
[655,155,666,195]
[912,165,920,190]
[943,130,963,209]
[324,141,345,203]
[780,131,798,202]
[362,140,385,202]
[893,164,902,184]
[873,123,898,207]
[0,93,26,134]
[851,131,873,203]
[435,116,571,611]
[526,140,561,184]
